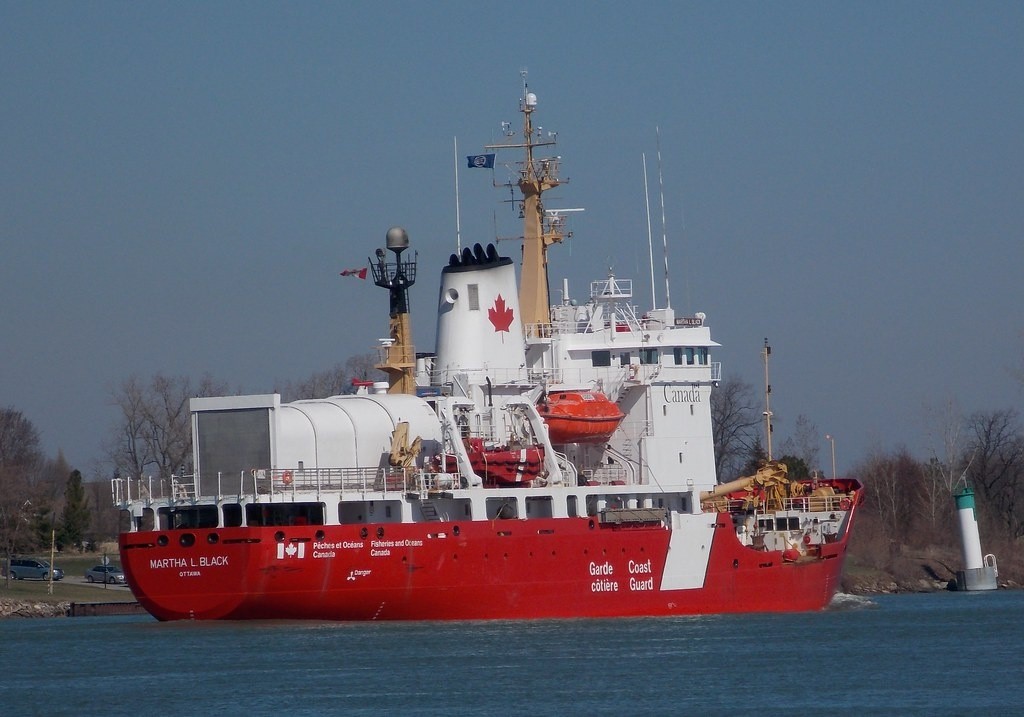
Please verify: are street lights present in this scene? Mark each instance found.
[825,434,836,480]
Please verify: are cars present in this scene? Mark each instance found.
[84,564,127,584]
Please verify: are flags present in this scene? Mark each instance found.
[467,153,496,169]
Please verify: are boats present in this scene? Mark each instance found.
[107,70,865,630]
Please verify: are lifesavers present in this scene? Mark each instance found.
[840,498,851,510]
[283,471,292,483]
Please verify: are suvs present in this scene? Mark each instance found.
[3,557,65,581]
